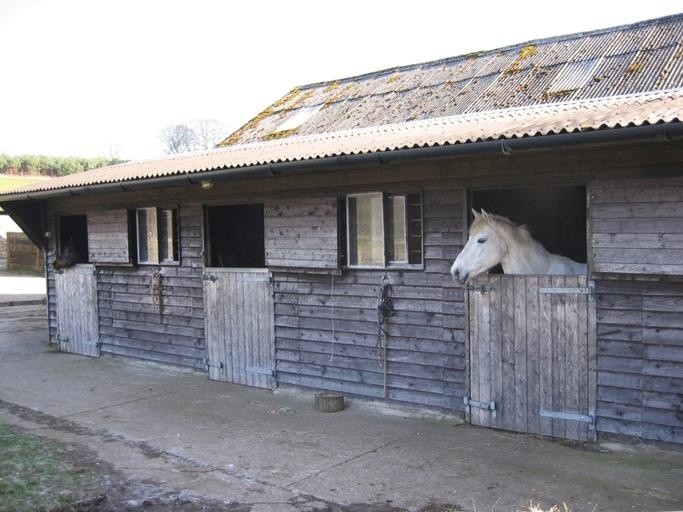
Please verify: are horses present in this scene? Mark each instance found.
[52,232,88,267]
[450,207,587,286]
